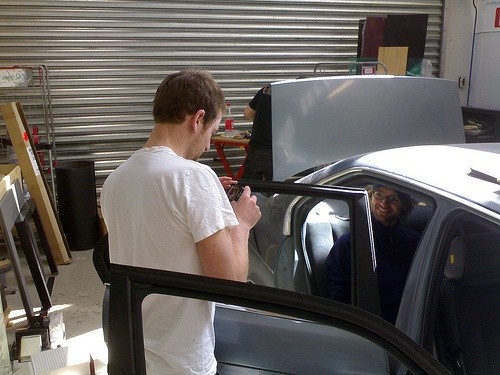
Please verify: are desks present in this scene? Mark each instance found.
[211,136,253,180]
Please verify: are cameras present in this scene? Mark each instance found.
[226,184,252,201]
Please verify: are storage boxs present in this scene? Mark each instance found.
[12,346,91,375]
[0,164,23,203]
[1,102,73,264]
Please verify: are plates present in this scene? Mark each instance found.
[216,132,225,136]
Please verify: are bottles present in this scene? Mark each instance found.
[223,103,235,138]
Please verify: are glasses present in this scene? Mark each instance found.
[373,191,400,203]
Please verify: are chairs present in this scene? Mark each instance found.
[441,233,500,375]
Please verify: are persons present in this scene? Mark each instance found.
[240,75,316,183]
[99,70,262,375]
[320,184,471,326]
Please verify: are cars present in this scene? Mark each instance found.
[106,75,500,375]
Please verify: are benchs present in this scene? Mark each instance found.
[308,220,350,272]
